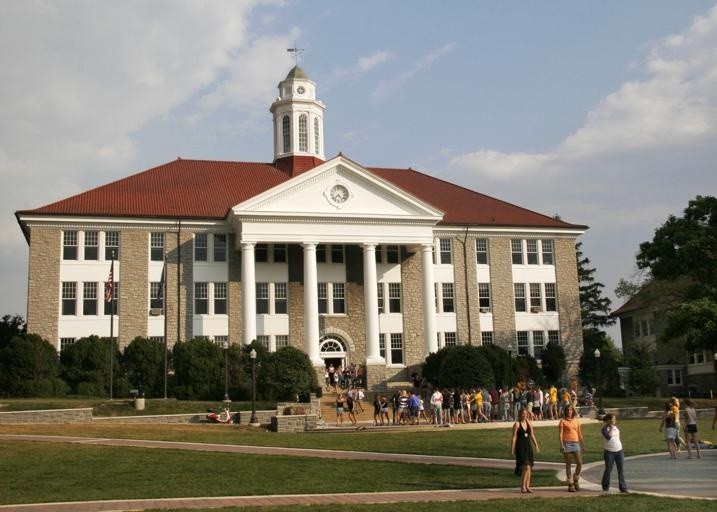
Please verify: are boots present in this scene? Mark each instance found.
[567,474,581,492]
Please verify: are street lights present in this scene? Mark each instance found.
[507,343,513,389]
[250,349,257,416]
[223,341,230,400]
[595,349,602,411]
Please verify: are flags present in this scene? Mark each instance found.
[156,264,165,301]
[105,259,115,304]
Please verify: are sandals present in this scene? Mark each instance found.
[521,487,533,493]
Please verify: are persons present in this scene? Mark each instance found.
[600,412,630,493]
[680,397,703,459]
[659,399,678,459]
[558,403,587,491]
[667,395,688,452]
[322,363,600,424]
[710,407,717,431]
[509,407,540,493]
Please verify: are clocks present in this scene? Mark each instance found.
[324,180,352,210]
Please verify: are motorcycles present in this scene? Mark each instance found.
[207,408,234,424]
